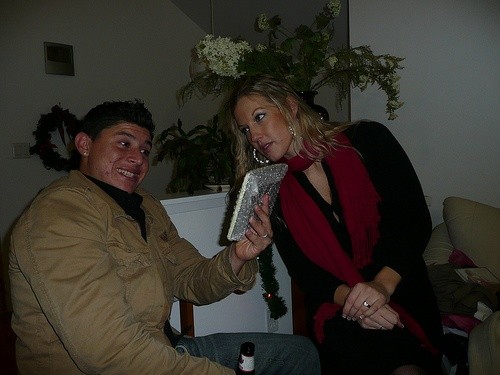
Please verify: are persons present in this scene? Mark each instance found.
[8,97,321,375]
[226,72,444,375]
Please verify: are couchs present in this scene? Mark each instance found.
[422,222,500,375]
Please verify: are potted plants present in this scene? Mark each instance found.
[165,116,231,189]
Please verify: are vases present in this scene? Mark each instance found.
[297,91,329,122]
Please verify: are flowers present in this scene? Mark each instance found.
[180,0,405,121]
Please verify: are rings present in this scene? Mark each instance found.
[260,234,267,238]
[378,325,382,330]
[363,301,371,308]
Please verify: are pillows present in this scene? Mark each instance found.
[443,196,500,277]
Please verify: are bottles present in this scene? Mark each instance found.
[235,341,255,375]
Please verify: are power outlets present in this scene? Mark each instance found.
[12,142,31,159]
